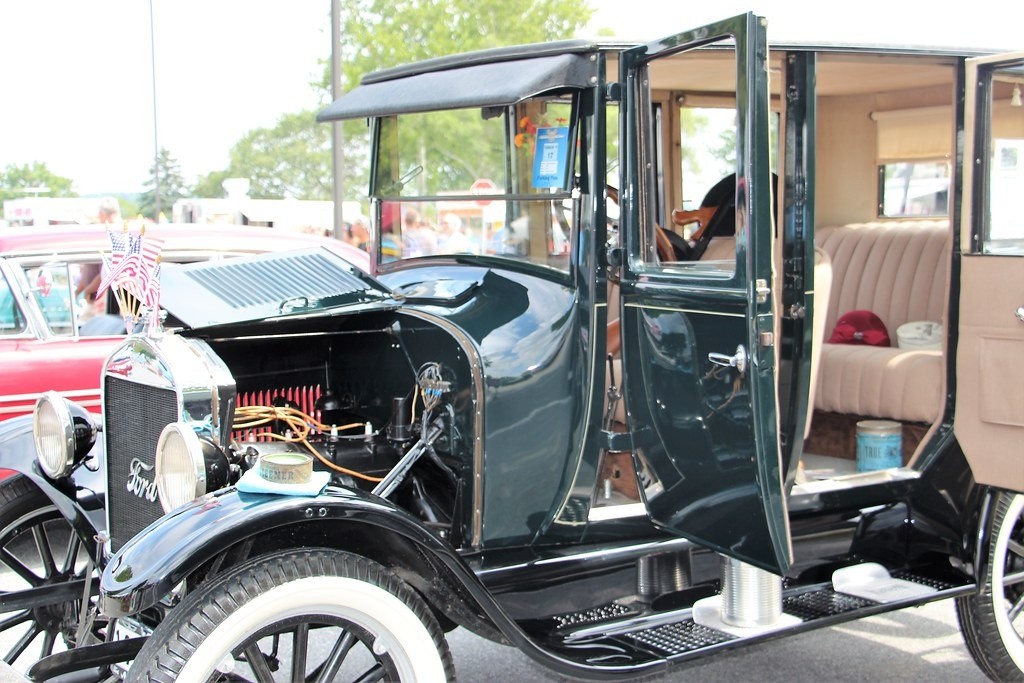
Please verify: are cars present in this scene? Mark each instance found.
[0,9,1024,683]
[0,227,368,568]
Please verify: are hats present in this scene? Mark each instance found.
[825,310,891,347]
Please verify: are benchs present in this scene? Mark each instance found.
[688,217,953,427]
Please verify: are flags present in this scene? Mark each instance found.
[97,223,161,307]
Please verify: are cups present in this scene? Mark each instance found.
[855,421,903,472]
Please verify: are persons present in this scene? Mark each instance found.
[402,209,428,256]
[437,214,469,255]
[343,222,353,241]
[75,263,101,304]
[352,218,370,251]
[420,218,434,244]
